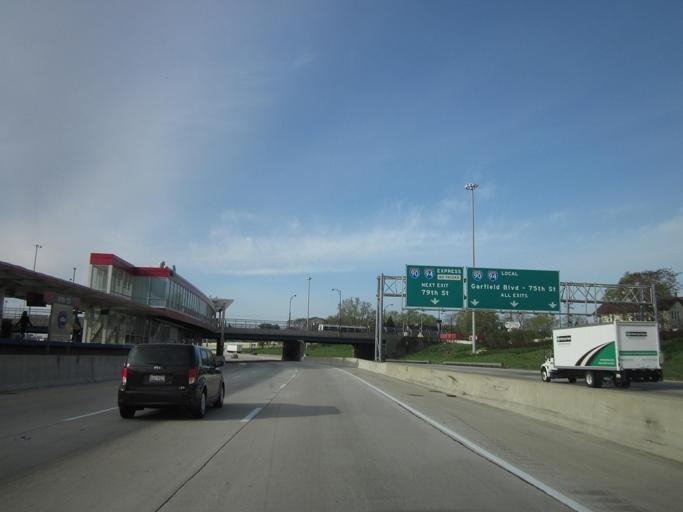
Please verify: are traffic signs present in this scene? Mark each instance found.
[467,268,560,312]
[406,266,463,308]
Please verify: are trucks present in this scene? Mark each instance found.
[537,321,666,390]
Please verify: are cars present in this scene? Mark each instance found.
[230,352,239,359]
[117,340,228,424]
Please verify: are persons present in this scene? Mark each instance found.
[16,311,33,338]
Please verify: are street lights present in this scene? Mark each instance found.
[331,287,342,332]
[463,182,480,354]
[288,293,297,328]
[305,276,312,328]
[33,244,43,272]
[73,267,77,283]
[383,303,394,326]
